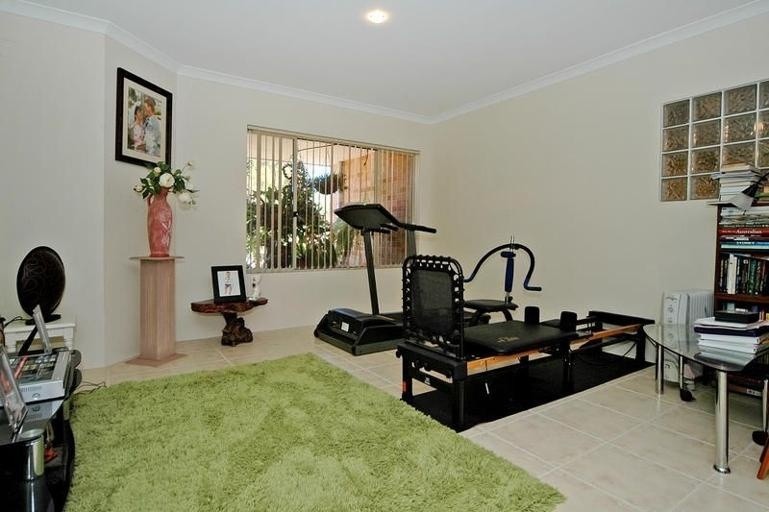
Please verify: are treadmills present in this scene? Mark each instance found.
[314,204,490,356]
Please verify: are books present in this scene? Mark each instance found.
[718,251,769,297]
[692,301,769,367]
[15,336,65,353]
[717,206,769,249]
[709,159,763,202]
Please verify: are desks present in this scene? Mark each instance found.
[191,298,268,346]
[0,346,82,512]
[642,323,768,473]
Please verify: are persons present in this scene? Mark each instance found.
[223,271,233,296]
[143,96,162,153]
[132,105,144,152]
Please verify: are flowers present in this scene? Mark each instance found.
[130,159,198,205]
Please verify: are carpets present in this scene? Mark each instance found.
[64,350,566,511]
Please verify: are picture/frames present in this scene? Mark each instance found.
[211,265,248,303]
[114,66,174,169]
[0,341,29,433]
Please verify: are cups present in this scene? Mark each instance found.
[19,429,45,482]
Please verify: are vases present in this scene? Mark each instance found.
[146,187,174,256]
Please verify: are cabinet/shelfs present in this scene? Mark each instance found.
[708,201,768,402]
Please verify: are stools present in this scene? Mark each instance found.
[2,314,79,353]
